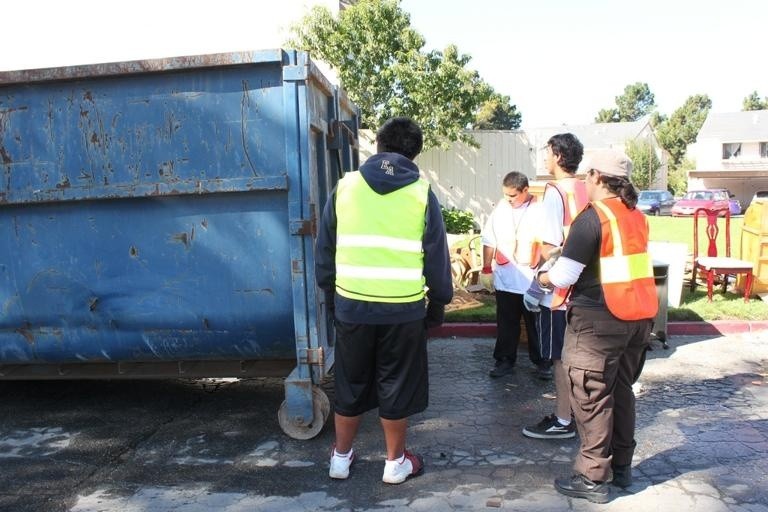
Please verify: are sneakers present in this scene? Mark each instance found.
[327,442,357,480]
[380,449,424,484]
[521,413,576,439]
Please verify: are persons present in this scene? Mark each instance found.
[521,141,662,505]
[516,132,593,441]
[313,116,454,486]
[480,171,553,381]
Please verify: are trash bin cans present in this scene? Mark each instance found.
[736,200,768,295]
[648,265,669,350]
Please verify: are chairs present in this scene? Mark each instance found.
[689,206,754,305]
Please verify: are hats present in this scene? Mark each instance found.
[582,147,635,179]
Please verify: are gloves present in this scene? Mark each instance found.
[423,300,446,329]
[481,265,495,292]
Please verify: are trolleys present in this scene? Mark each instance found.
[647,258,673,353]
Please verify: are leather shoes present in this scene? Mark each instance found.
[610,464,633,487]
[553,473,610,504]
[487,361,513,376]
[536,361,553,379]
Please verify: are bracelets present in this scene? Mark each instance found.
[482,267,492,274]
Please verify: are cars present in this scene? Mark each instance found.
[636,188,743,218]
